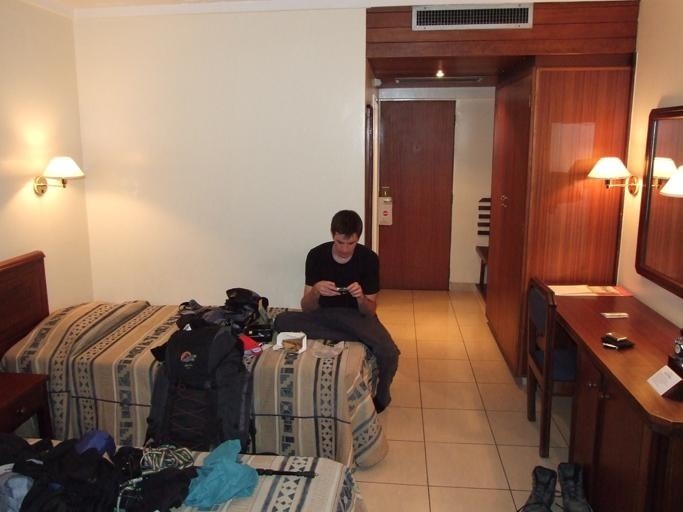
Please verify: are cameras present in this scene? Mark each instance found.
[337,287,348,295]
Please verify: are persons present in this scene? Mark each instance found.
[275,210,401,414]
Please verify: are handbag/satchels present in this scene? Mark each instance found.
[176,288,267,330]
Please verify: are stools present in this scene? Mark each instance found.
[475,245,488,292]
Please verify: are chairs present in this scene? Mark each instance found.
[526,275,583,458]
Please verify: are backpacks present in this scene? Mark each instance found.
[143,331,253,452]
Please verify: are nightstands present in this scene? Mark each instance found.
[0,372,56,440]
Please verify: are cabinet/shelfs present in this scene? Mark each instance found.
[539,281,683,512]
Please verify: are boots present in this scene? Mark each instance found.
[523,466,557,511]
[558,462,592,512]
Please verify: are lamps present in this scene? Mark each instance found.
[31,155,87,196]
[586,156,639,196]
[650,157,683,198]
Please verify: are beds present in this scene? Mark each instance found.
[0,438,346,512]
[0,249,368,467]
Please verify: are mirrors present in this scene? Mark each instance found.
[635,104,683,299]
